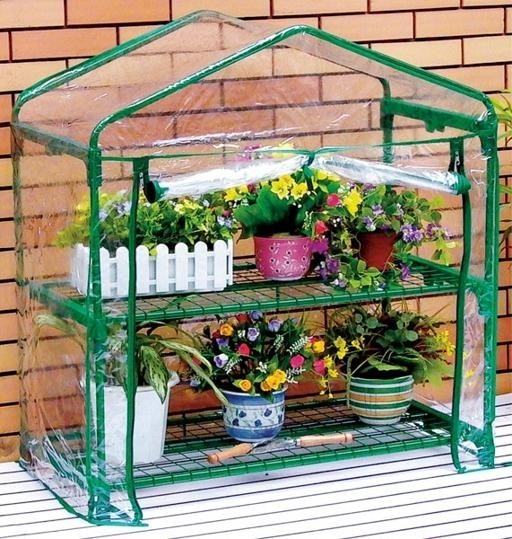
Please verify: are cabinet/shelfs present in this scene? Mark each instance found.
[14,5,498,528]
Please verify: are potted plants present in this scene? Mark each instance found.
[29,295,221,473]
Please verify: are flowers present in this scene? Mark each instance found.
[313,301,456,399]
[322,182,450,292]
[54,172,244,245]
[180,307,331,400]
[228,140,342,238]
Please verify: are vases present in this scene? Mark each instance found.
[354,227,401,273]
[71,245,235,298]
[252,237,313,284]
[342,370,413,428]
[217,386,284,444]
